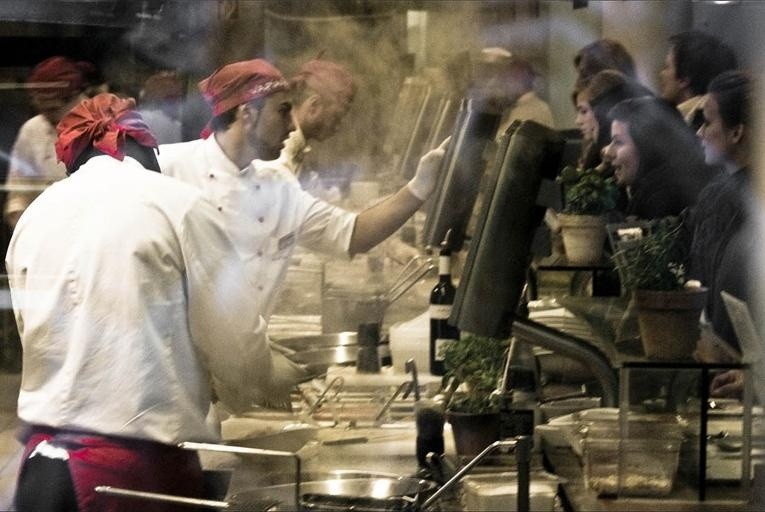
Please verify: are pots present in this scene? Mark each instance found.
[93,440,440,512]
[266,252,438,376]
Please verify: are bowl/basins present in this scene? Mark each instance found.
[534,406,632,448]
[348,180,381,209]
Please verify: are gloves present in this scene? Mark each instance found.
[409,134,451,201]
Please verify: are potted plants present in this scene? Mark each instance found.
[434,329,515,465]
[547,166,621,266]
[611,223,713,361]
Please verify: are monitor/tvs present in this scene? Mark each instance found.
[383,78,458,216]
[421,98,502,254]
[447,120,567,345]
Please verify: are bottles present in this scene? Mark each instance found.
[428,247,460,376]
